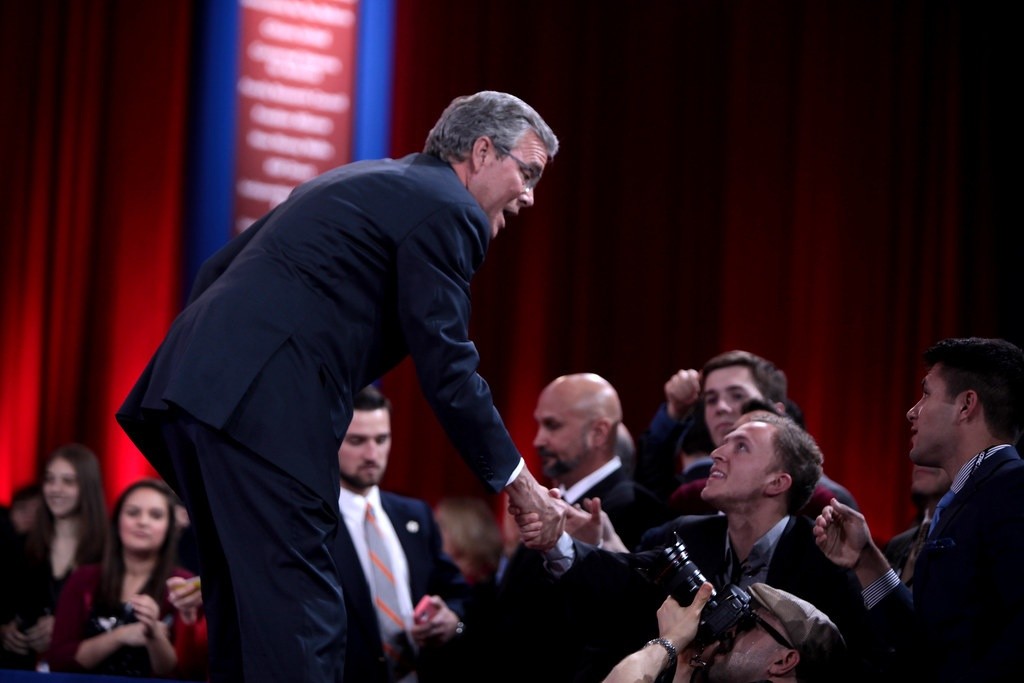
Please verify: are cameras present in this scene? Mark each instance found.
[637,529,752,647]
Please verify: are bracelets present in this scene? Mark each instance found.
[645,638,676,667]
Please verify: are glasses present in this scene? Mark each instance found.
[499,146,542,192]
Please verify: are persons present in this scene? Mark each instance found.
[338,334,1024,683]
[0,439,210,683]
[114,91,566,683]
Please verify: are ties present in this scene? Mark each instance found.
[901,522,931,595]
[928,490,956,540]
[364,504,415,679]
